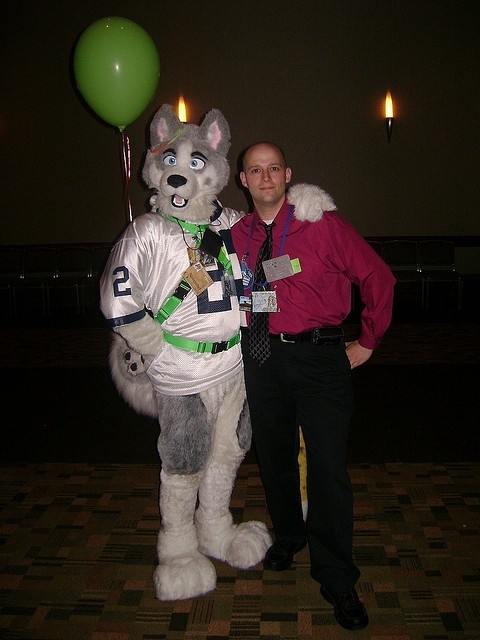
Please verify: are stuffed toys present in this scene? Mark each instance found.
[99,102,338,603]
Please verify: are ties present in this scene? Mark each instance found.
[248,223,276,365]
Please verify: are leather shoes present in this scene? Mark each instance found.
[319,581,371,631]
[265,534,308,569]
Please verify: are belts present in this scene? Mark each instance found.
[270,331,344,346]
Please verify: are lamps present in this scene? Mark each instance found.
[384,90,394,143]
[177,95,187,124]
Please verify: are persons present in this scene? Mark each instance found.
[228,140,399,632]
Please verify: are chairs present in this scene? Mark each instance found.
[384,240,425,308]
[455,247,480,310]
[11,247,55,319]
[0,247,22,317]
[419,240,463,311]
[82,246,114,320]
[352,241,381,309]
[45,246,90,319]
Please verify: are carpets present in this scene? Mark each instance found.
[0,359,479,464]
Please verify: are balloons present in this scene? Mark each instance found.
[71,15,161,134]
